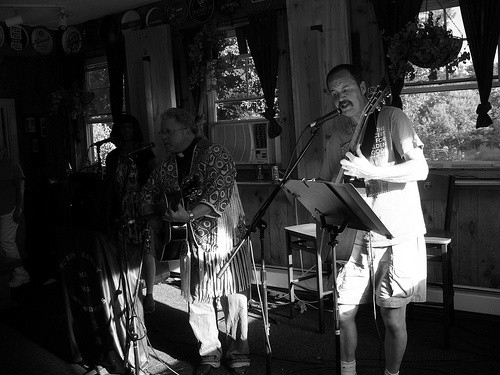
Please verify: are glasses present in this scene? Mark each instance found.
[158,128,187,138]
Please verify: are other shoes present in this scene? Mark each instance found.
[197,364,216,375]
[233,366,248,375]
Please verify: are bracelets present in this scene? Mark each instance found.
[187,210,195,222]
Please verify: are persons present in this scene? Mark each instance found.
[138,108,256,375]
[106,113,171,312]
[318,63,429,375]
[0,158,30,288]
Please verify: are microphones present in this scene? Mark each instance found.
[124,142,156,158]
[306,108,342,128]
[90,136,117,147]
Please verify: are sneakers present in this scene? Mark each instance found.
[6,270,31,288]
[143,294,155,314]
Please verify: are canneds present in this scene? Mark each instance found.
[271,165,280,181]
[256,165,264,180]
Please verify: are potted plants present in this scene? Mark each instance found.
[378,13,470,83]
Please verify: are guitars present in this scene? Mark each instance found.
[155,174,204,263]
[316,85,391,262]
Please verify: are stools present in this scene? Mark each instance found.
[283,222,339,335]
[407,236,452,352]
